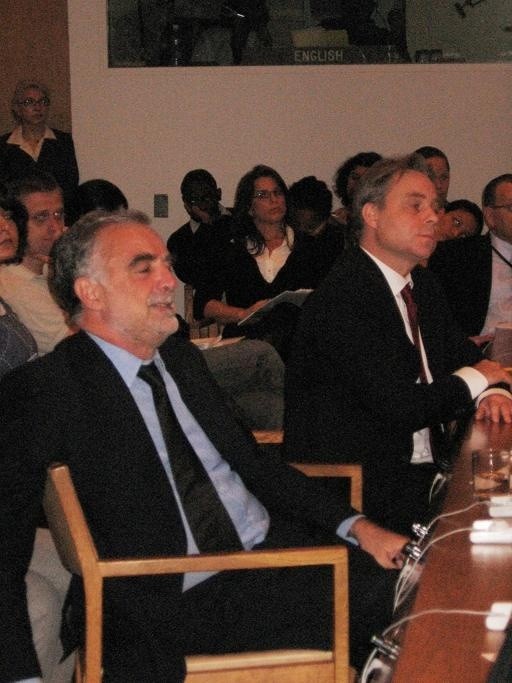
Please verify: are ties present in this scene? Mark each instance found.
[137,362,249,579]
[402,286,449,474]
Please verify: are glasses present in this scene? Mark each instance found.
[12,97,49,109]
[249,187,282,202]
[492,202,510,213]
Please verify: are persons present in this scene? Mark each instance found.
[0,80,80,204]
[413,146,512,367]
[332,154,383,208]
[1,175,288,428]
[279,158,512,544]
[0,208,422,683]
[167,163,348,362]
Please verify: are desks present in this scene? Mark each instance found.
[389,328,511,682]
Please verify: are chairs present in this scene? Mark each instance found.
[42,463,360,682]
[198,339,365,514]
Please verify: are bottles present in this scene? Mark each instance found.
[375,39,392,63]
[171,24,182,57]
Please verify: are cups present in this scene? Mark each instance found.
[472,448,512,500]
[414,48,442,63]
